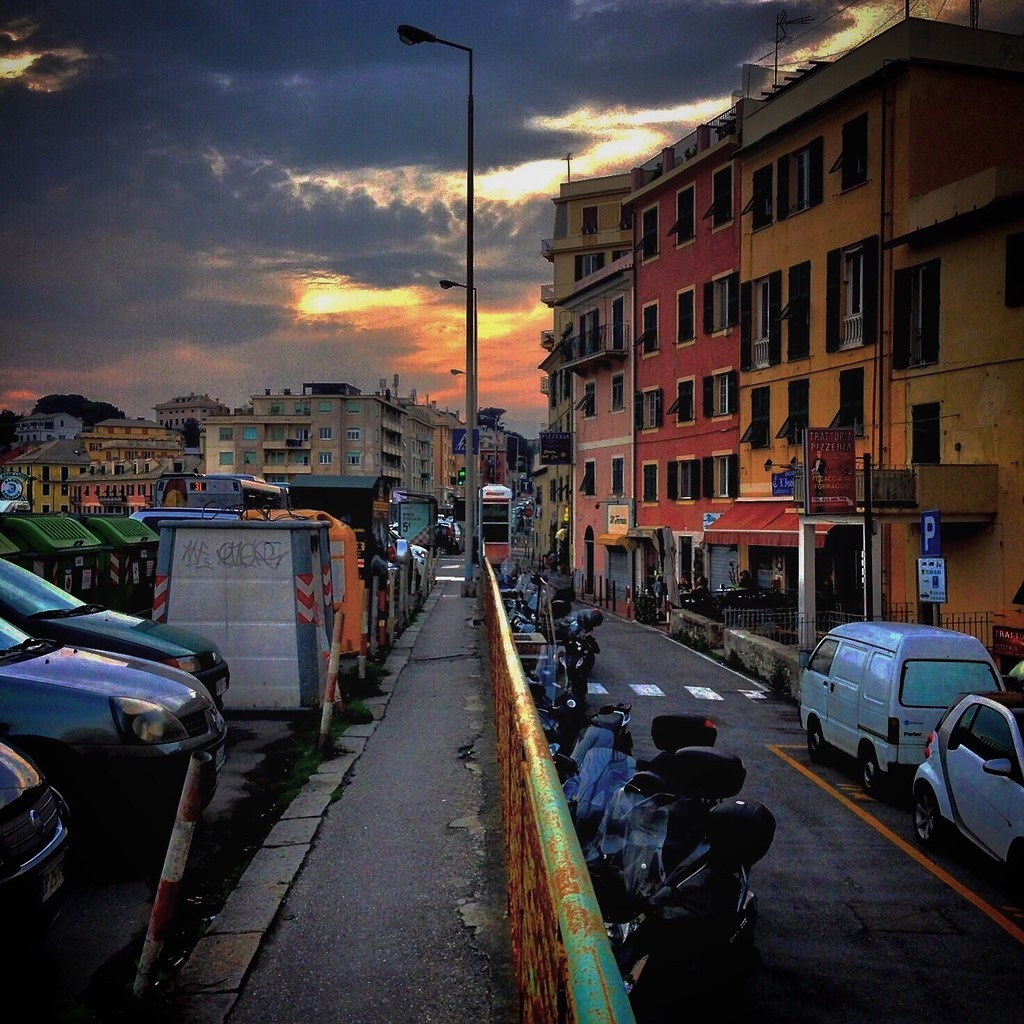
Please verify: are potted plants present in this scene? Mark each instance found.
[633,591,656,625]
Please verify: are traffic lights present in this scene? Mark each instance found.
[458,470,465,487]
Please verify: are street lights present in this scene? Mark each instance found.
[436,276,484,564]
[517,454,529,482]
[449,368,478,536]
[504,433,519,534]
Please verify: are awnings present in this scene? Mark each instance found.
[555,529,567,540]
[704,503,836,549]
[625,526,661,553]
[323,511,372,541]
[596,533,626,548]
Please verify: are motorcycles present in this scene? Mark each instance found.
[490,555,777,1024]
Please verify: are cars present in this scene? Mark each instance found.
[382,513,464,591]
[0,743,75,957]
[511,496,533,530]
[912,692,1024,897]
[0,615,229,855]
[0,554,231,716]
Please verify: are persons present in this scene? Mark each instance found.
[547,547,566,574]
[647,573,665,614]
[689,575,711,611]
[738,570,754,596]
[678,575,691,591]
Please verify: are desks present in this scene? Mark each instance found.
[717,587,747,597]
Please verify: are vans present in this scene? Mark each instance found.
[795,620,1008,800]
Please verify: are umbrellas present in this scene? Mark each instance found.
[663,526,683,623]
